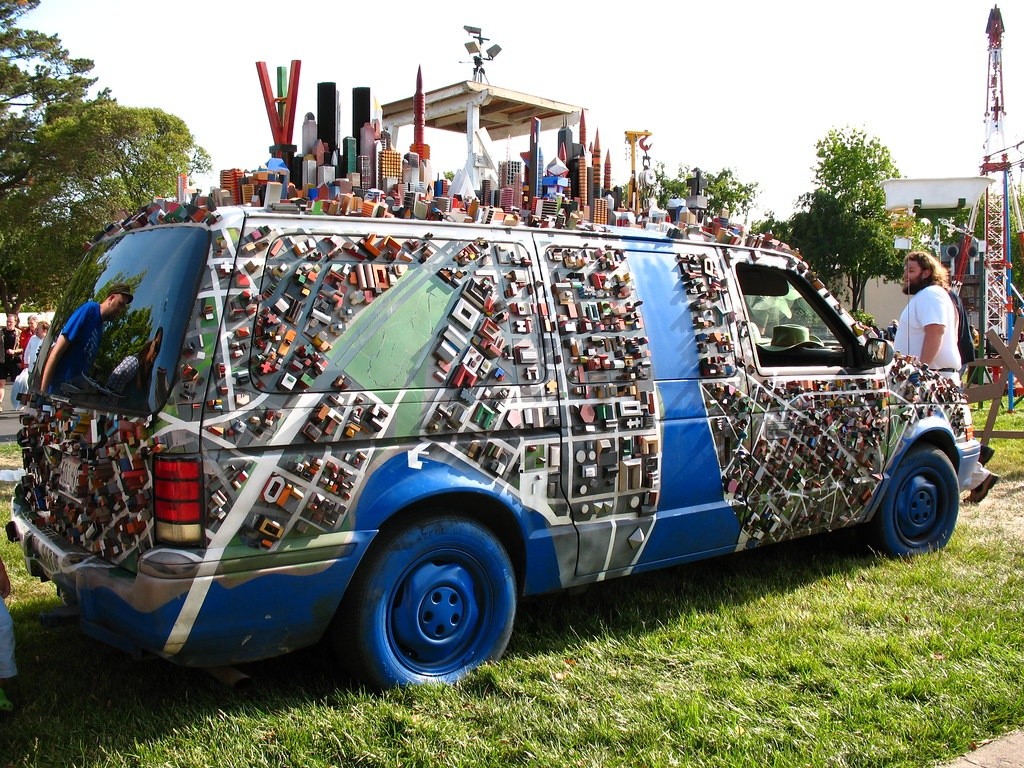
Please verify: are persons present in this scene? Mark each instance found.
[0,558,25,711]
[41,283,134,397]
[892,252,998,504]
[101,327,164,408]
[0,314,50,411]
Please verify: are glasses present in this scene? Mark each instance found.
[153,341,161,352]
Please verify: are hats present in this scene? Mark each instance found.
[107,284,134,304]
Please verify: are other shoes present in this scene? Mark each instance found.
[963,473,998,504]
[977,445,995,467]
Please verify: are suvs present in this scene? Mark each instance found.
[8,206,982,694]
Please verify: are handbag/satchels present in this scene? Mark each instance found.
[11,368,30,411]
